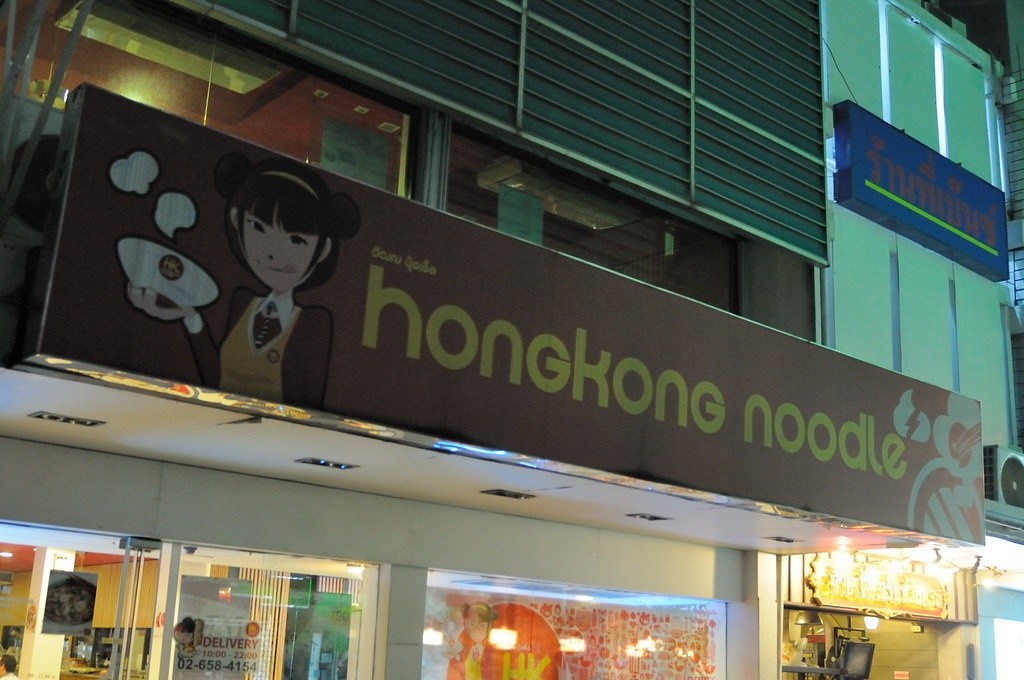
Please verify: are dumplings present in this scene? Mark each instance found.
[45,585,95,623]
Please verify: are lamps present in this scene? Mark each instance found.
[29,19,69,109]
[795,610,823,626]
[911,621,922,632]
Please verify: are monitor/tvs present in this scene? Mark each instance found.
[838,641,875,680]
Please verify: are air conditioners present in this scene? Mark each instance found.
[984,444,1024,509]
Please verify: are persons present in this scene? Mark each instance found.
[0,654,19,680]
